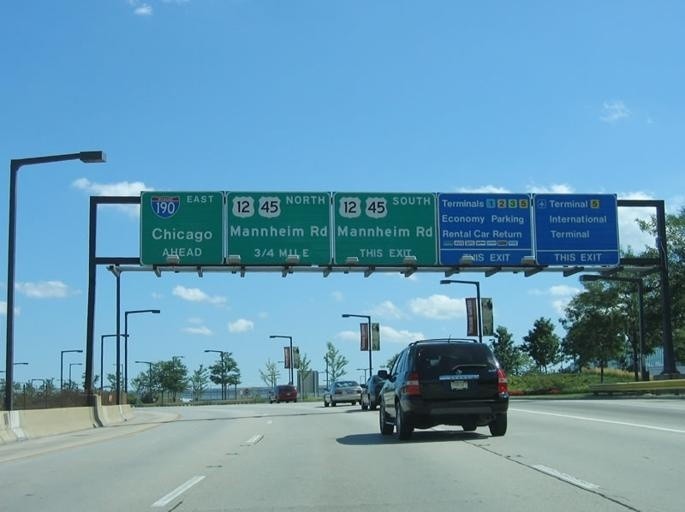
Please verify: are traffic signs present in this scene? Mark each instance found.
[333,193,438,265]
[227,193,331,267]
[140,192,225,269]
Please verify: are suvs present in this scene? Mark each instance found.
[377,339,509,440]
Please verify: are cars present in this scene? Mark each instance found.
[360,375,388,410]
[323,381,363,407]
[268,385,297,404]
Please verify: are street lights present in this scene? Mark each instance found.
[342,314,372,378]
[13,363,28,365]
[124,310,160,392]
[579,274,647,380]
[135,361,151,387]
[5,151,106,409]
[205,350,223,401]
[61,349,83,392]
[440,280,482,343]
[270,335,293,384]
[101,334,129,391]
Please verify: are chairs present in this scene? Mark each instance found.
[436,356,453,369]
